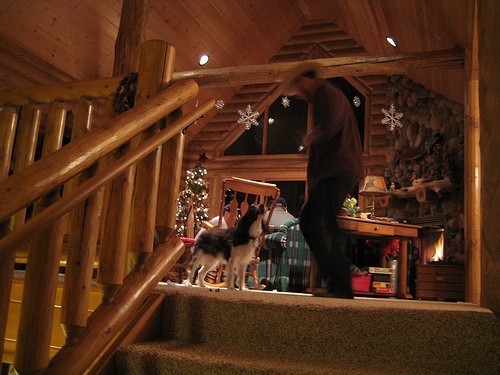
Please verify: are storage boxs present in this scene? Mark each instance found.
[352,267,396,293]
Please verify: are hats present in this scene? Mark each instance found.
[280,60,323,95]
[271,198,285,207]
[223,204,240,211]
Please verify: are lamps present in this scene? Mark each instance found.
[359,176,389,220]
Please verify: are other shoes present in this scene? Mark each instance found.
[312,289,354,299]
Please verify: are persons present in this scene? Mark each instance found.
[262,197,296,233]
[191,204,241,253]
[285,62,362,300]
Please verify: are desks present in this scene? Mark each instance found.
[307,215,422,300]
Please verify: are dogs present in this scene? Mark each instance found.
[189,204,266,292]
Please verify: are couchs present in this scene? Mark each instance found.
[244,218,311,292]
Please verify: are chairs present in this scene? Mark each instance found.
[167,177,280,289]
